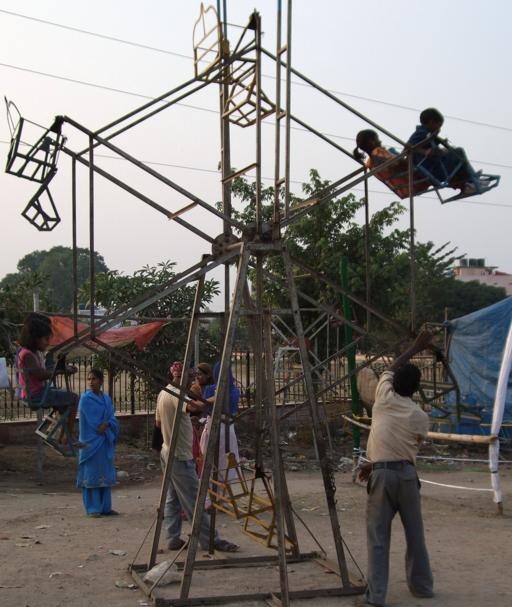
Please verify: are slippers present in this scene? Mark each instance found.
[215,540,240,552]
[58,441,92,449]
[89,512,100,517]
[109,510,120,515]
[168,540,187,550]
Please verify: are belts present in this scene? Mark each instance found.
[369,460,412,471]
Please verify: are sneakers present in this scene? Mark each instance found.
[461,169,490,195]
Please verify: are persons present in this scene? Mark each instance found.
[181,361,214,521]
[356,327,435,606]
[76,367,121,517]
[352,129,483,198]
[402,106,491,196]
[154,359,240,551]
[17,316,92,449]
[14,310,80,414]
[200,359,242,512]
